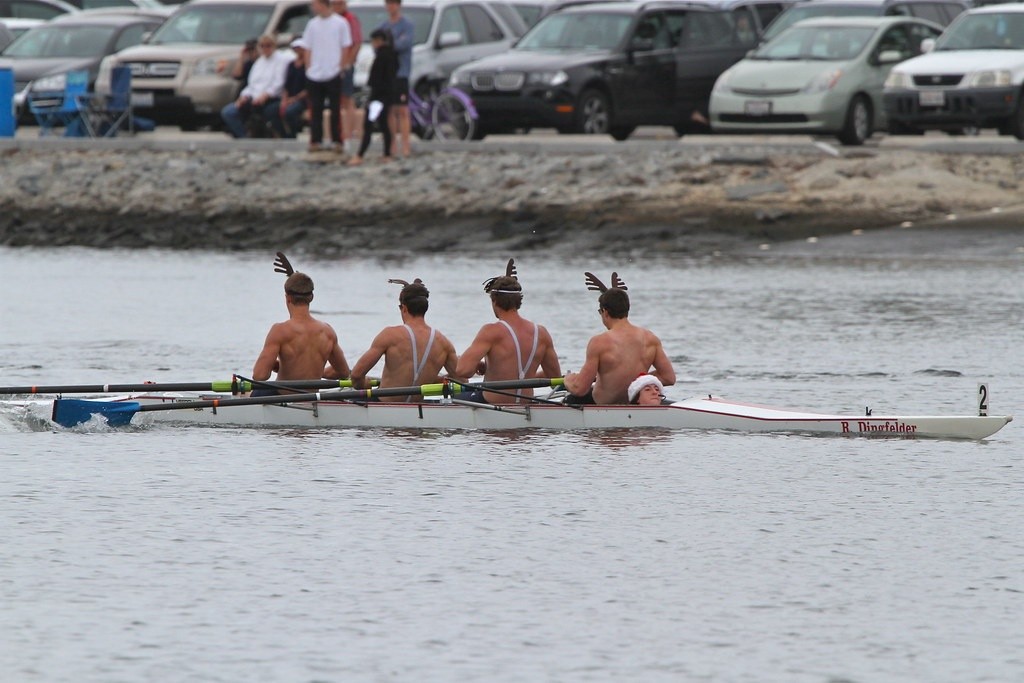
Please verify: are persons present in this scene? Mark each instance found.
[236,272,349,397]
[628,372,664,405]
[350,283,468,402]
[346,28,400,165]
[220,0,362,153]
[453,276,561,404]
[379,0,411,155]
[561,286,676,404]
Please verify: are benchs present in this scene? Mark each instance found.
[302,109,365,140]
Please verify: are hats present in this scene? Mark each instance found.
[627,372,664,403]
[243,39,257,52]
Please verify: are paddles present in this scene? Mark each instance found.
[52,375,565,430]
[1,379,379,398]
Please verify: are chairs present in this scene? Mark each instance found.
[27,65,91,136]
[75,67,132,137]
[639,22,655,38]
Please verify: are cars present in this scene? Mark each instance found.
[0,0,973,149]
[705,14,948,150]
[880,1,1024,142]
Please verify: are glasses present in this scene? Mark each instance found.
[598,307,607,314]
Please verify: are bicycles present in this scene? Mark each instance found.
[404,81,479,145]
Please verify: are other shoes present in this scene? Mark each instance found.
[347,156,364,166]
[381,156,398,162]
[309,141,322,151]
[332,140,343,153]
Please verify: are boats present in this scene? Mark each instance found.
[0,365,1017,448]
